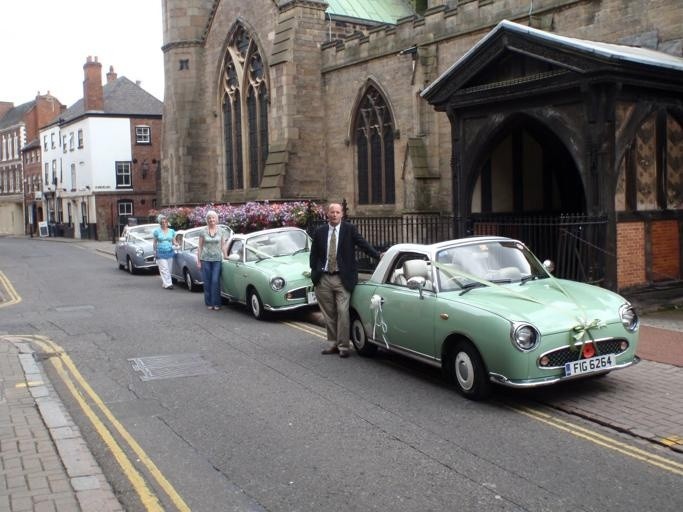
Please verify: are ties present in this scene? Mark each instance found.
[327,228,337,275]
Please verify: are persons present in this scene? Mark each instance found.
[152,214,178,289]
[309,204,385,357]
[197,210,229,309]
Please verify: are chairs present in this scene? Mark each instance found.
[397,260,433,294]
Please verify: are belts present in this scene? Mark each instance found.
[322,271,340,276]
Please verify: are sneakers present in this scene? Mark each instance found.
[320,344,338,355]
[339,351,351,358]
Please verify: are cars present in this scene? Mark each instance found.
[170,223,236,293]
[114,223,161,275]
[347,232,642,400]
[219,226,318,322]
